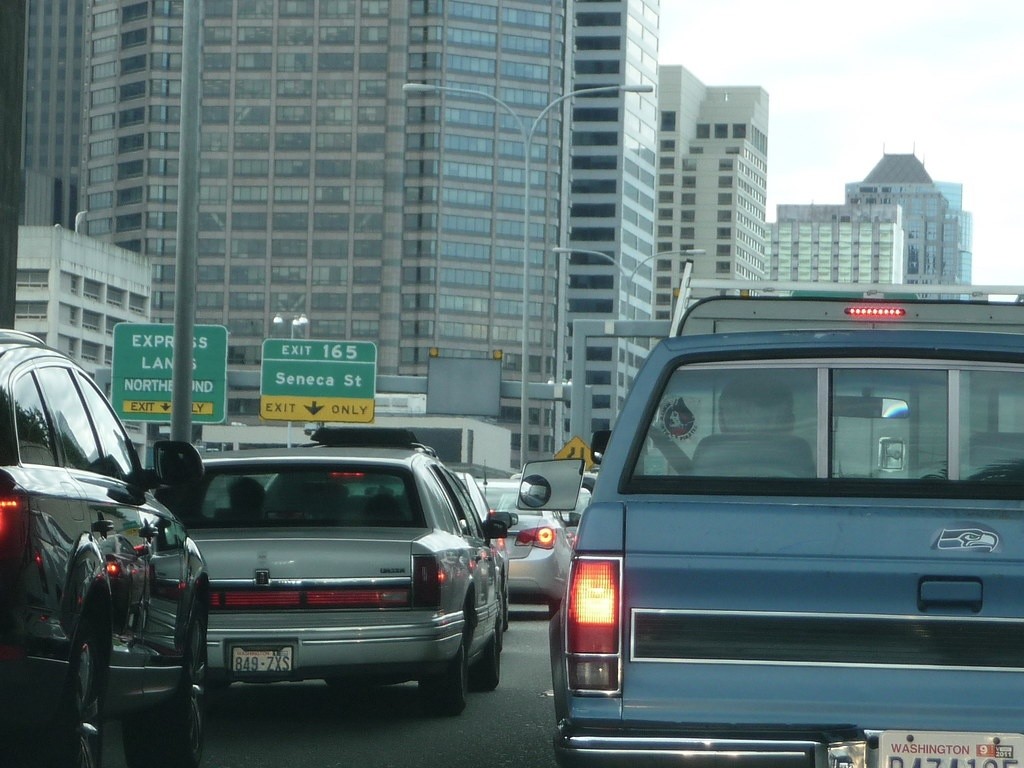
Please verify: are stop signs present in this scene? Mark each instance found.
[256,334,379,424]
[102,323,227,427]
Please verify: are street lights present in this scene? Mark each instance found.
[401,77,657,463]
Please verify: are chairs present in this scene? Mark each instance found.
[690,371,819,480]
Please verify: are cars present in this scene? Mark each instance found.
[0,326,209,767]
[466,466,578,627]
[169,438,519,717]
[514,266,1021,768]
